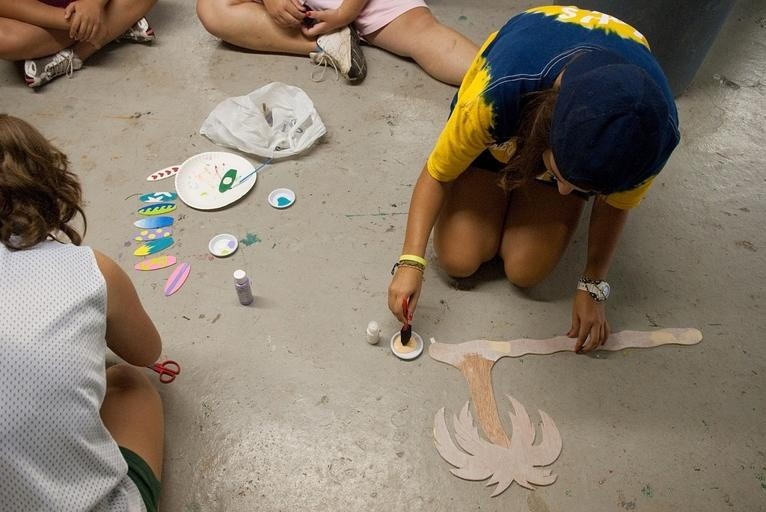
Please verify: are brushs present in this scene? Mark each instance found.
[401,297,411,345]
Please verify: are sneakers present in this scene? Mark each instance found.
[308,23,368,84]
[114,15,155,44]
[20,49,84,89]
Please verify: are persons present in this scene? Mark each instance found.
[1,0,157,89]
[0,112,166,512]
[196,0,482,88]
[387,4,681,353]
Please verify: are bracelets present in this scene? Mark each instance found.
[390,254,427,275]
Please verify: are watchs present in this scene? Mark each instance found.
[576,277,612,302]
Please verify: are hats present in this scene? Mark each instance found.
[550,51,680,195]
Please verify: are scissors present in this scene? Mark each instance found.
[147,360,180,383]
